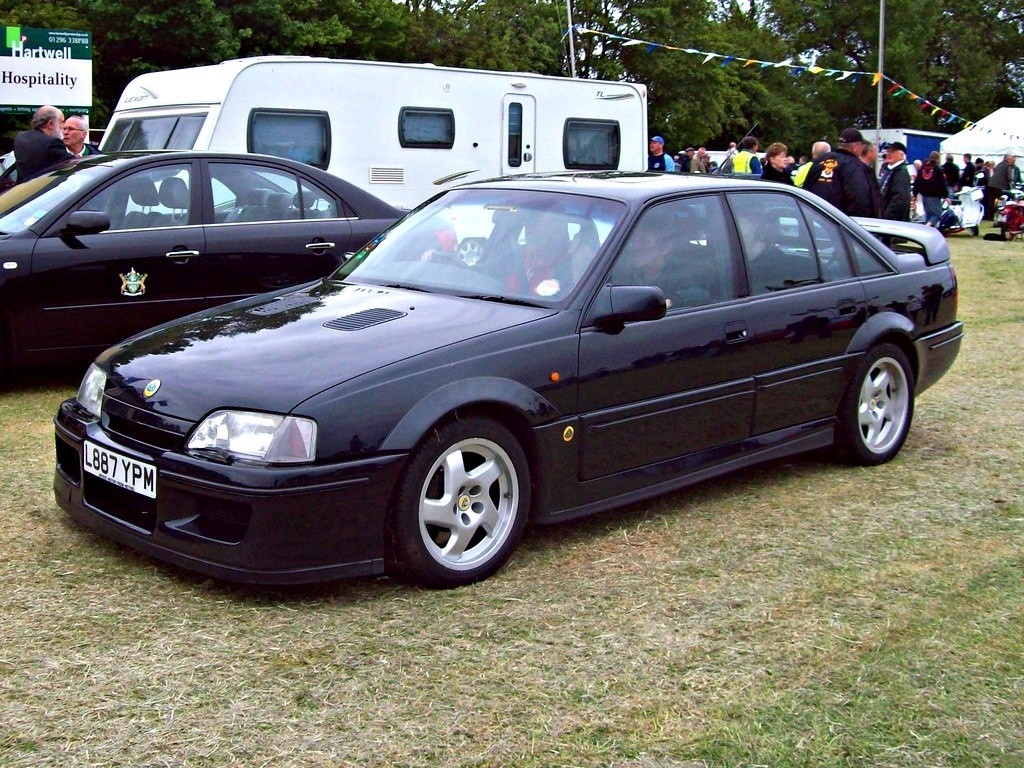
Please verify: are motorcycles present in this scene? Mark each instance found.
[992,182,1024,240]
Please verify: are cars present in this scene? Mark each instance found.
[55,174,964,589]
[0,155,454,388]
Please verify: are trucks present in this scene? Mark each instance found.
[97,56,648,235]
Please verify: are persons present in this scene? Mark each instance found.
[681,137,1024,241]
[63,115,101,158]
[800,128,876,218]
[420,202,791,307]
[647,136,676,173]
[14,105,69,185]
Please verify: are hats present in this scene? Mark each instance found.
[685,147,694,152]
[883,142,907,154]
[838,127,872,145]
[649,136,664,145]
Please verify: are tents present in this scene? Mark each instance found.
[940,107,1024,181]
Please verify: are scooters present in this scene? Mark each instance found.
[938,172,985,236]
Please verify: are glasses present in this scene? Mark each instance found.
[63,126,84,131]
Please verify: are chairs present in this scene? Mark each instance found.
[119,176,190,229]
[664,201,710,256]
[233,188,322,222]
[766,206,818,261]
[565,212,601,286]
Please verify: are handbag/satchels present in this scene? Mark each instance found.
[938,205,959,231]
